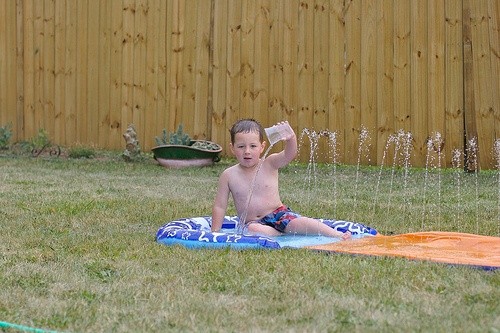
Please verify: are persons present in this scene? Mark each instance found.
[211,118,351,242]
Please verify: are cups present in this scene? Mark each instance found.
[264,122,294,145]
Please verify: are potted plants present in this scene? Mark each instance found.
[151,121,224,168]
